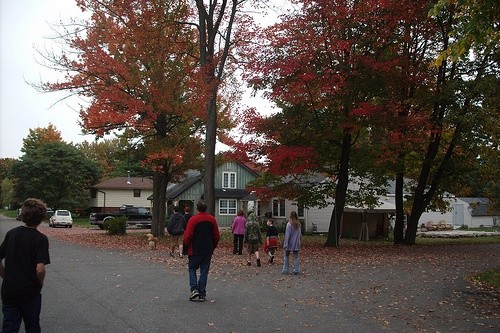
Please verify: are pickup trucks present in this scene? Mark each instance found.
[90,206,154,230]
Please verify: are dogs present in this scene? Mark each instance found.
[147,234,158,250]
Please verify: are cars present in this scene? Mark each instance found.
[16,208,22,221]
[49,210,73,228]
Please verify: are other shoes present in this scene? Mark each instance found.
[256,258,261,267]
[270,255,274,263]
[170,251,174,256]
[179,254,183,258]
[247,262,251,266]
[189,289,199,299]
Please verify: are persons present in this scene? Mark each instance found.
[182,200,220,302]
[0,198,51,333]
[184,207,192,222]
[264,219,278,263]
[245,213,261,267]
[232,210,246,255]
[281,211,301,275]
[167,206,186,257]
